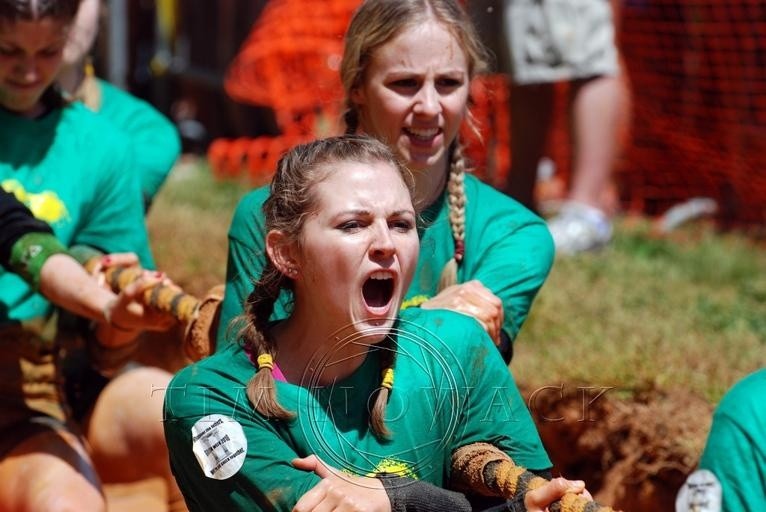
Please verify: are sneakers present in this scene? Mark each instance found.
[547,201,613,252]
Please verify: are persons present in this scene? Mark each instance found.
[0,0,187,511]
[673,363,766,512]
[162,132,596,512]
[56,0,184,219]
[212,0,556,368]
[464,0,633,254]
